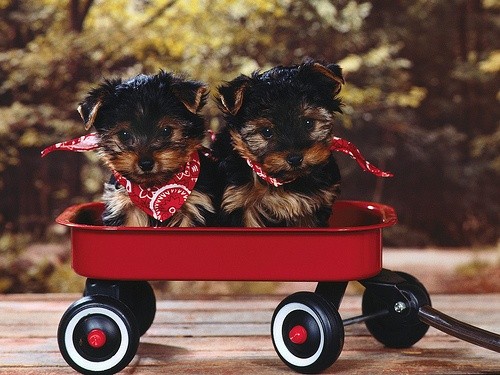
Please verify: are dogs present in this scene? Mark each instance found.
[76,61,346,229]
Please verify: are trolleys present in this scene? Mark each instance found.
[56,183,500,374]
